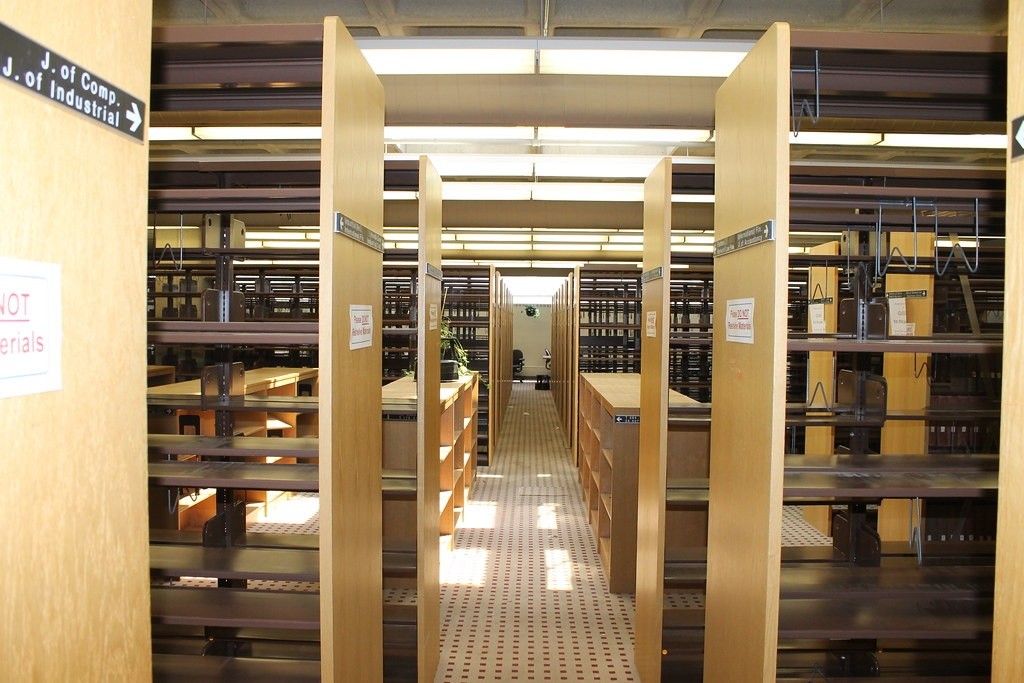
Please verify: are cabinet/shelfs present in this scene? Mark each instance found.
[151,16,1024,683]
